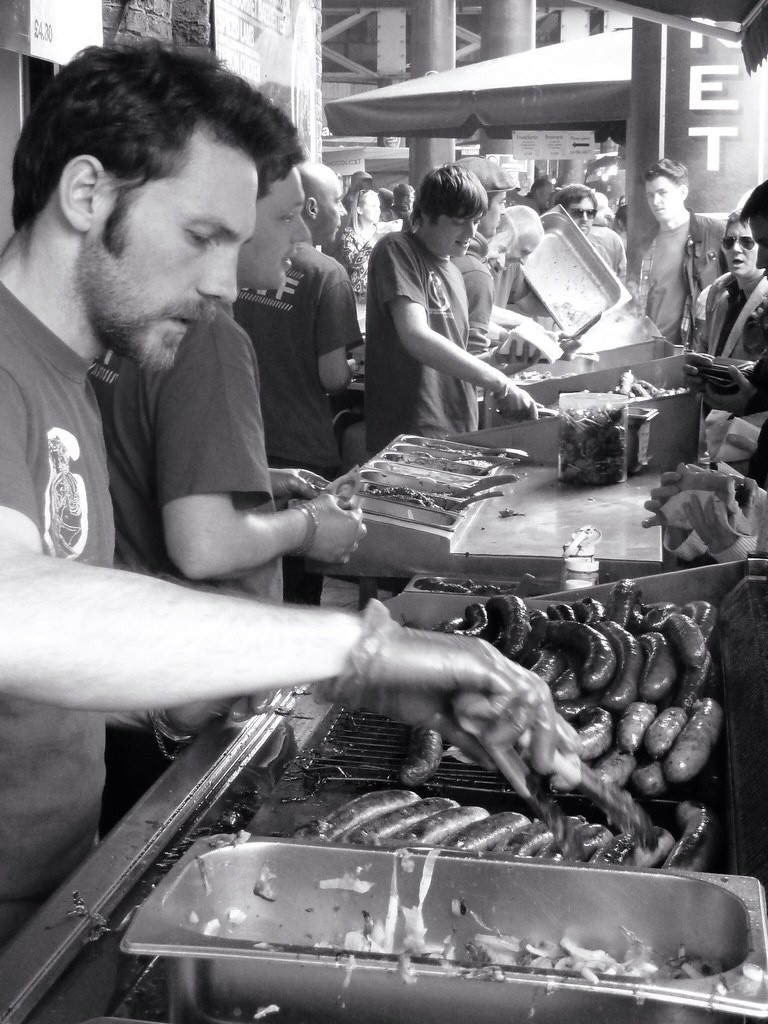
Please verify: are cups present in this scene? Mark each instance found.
[558,392,631,484]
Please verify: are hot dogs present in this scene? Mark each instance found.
[674,462,762,535]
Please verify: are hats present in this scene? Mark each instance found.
[455,157,517,193]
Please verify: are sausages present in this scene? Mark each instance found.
[425,578,724,794]
[400,726,442,785]
[614,369,661,399]
[294,789,718,872]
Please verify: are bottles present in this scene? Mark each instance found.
[559,524,602,592]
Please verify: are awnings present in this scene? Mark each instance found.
[549,0,768,73]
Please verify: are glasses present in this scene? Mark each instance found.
[568,207,598,220]
[722,235,757,251]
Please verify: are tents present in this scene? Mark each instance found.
[327,25,767,139]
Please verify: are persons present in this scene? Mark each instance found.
[0,34,586,952]
[95,154,768,850]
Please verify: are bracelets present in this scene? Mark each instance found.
[291,504,321,556]
[491,377,513,401]
[150,723,186,761]
